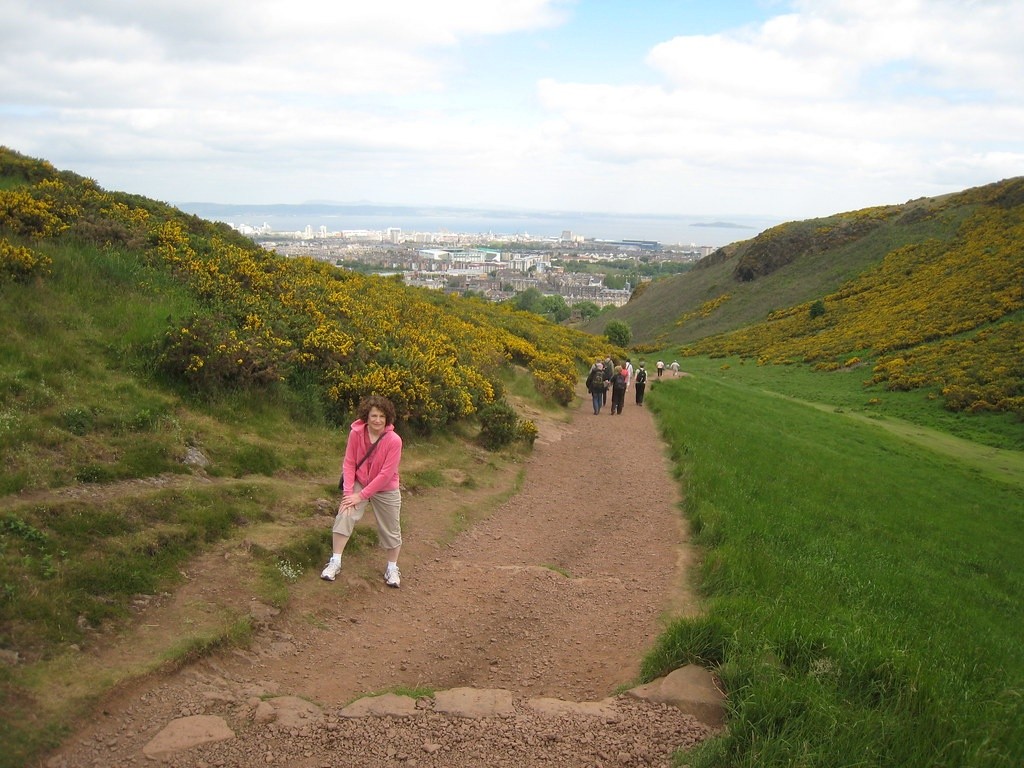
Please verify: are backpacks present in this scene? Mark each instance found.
[636,368,646,383]
[614,377,625,390]
[603,359,612,378]
[592,372,603,389]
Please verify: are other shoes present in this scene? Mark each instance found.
[639,402,643,406]
[594,411,598,415]
[611,410,615,415]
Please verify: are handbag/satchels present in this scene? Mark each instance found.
[338,471,343,490]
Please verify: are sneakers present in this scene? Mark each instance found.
[320,557,341,580]
[384,563,401,588]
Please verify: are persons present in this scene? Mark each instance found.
[634,362,647,406]
[656,359,664,377]
[321,397,402,588]
[616,361,628,406]
[590,358,633,406]
[671,360,679,376]
[586,363,607,415]
[608,366,626,415]
[666,363,670,369]
